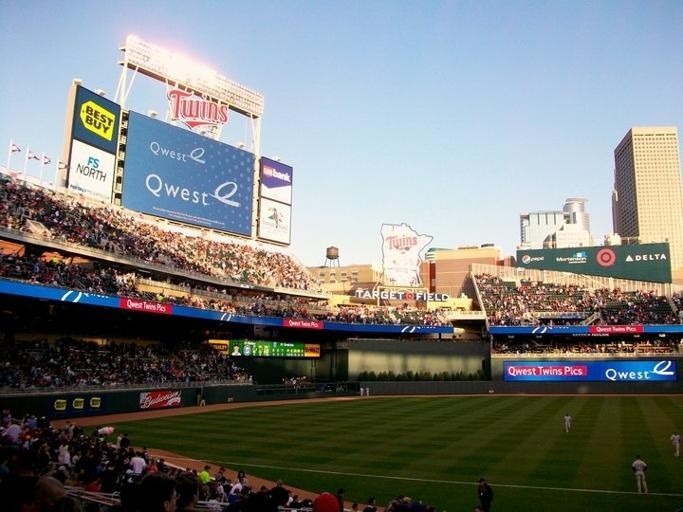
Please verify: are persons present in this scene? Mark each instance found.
[291,375,309,388]
[1,335,255,390]
[336,383,346,392]
[1,408,440,512]
[669,431,681,458]
[1,170,453,324]
[478,478,493,512]
[632,455,648,494]
[563,413,572,433]
[474,272,683,356]
[196,390,204,405]
[361,386,370,396]
[473,505,481,512]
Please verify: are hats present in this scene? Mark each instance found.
[479,478,484,483]
[368,497,375,504]
